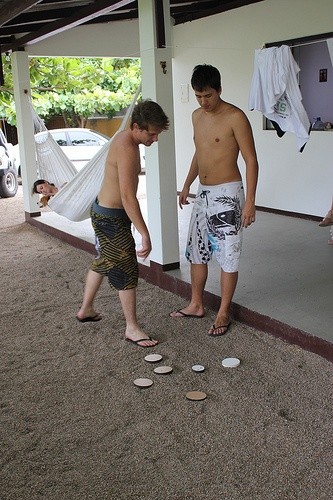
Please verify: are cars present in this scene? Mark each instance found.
[0,127,19,198]
[14,128,112,185]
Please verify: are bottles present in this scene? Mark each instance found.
[312,117,326,129]
[327,229,333,245]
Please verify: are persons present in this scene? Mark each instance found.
[75,100,169,348]
[31,179,93,222]
[170,63,259,337]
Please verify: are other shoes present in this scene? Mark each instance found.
[318,218,333,227]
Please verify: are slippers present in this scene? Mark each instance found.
[169,309,204,318]
[76,312,102,322]
[208,322,232,336]
[125,336,159,347]
